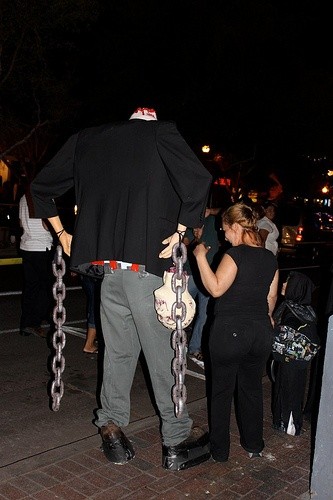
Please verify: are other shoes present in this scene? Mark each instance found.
[192,351,202,360]
[82,347,99,353]
[24,326,47,338]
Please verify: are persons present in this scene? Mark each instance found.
[175,191,232,371]
[254,203,280,261]
[28,106,212,471]
[18,171,59,337]
[267,271,319,436]
[79,274,101,352]
[192,202,280,463]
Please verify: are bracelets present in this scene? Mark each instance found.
[56,229,64,238]
